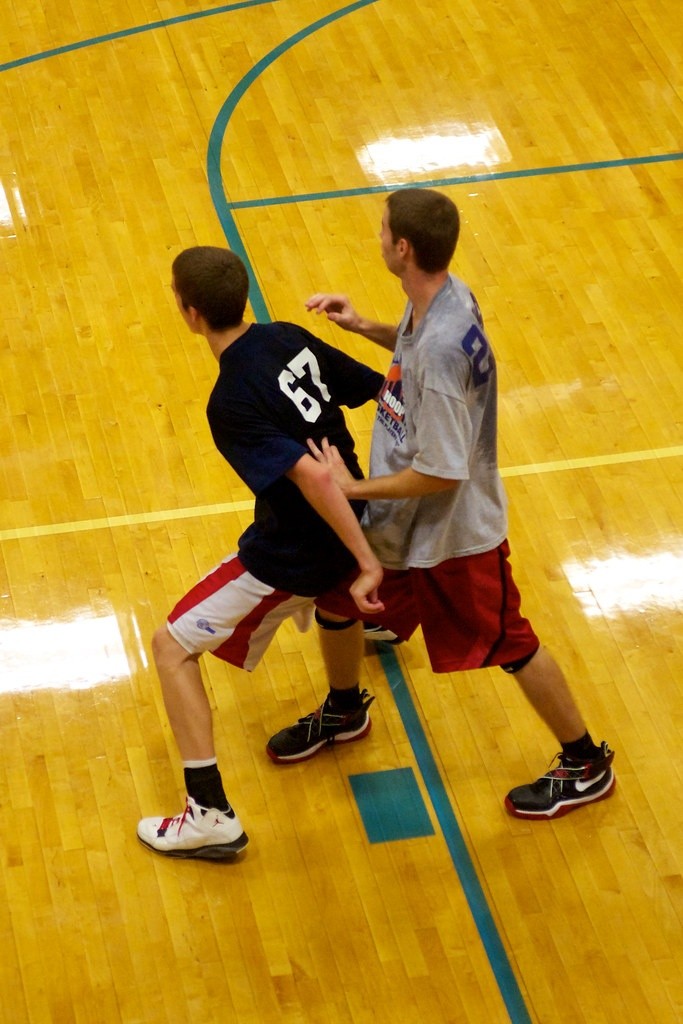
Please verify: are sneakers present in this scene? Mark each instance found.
[136,797,249,859]
[504,742,616,821]
[362,620,398,641]
[266,689,375,765]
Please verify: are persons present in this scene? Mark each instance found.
[302,186,615,819]
[136,245,388,858]
